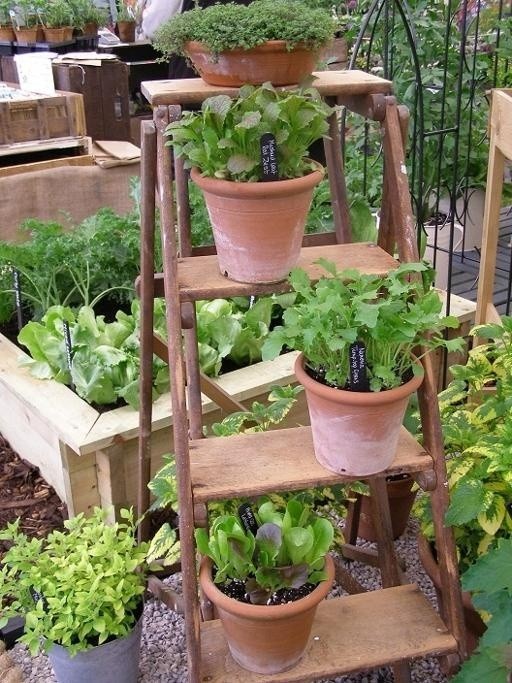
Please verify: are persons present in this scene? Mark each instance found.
[133,0,183,42]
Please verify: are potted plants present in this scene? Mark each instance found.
[0,504,167,682]
[1,2,138,45]
[196,495,337,677]
[164,76,345,286]
[280,257,512,477]
[149,0,345,86]
[345,475,419,543]
